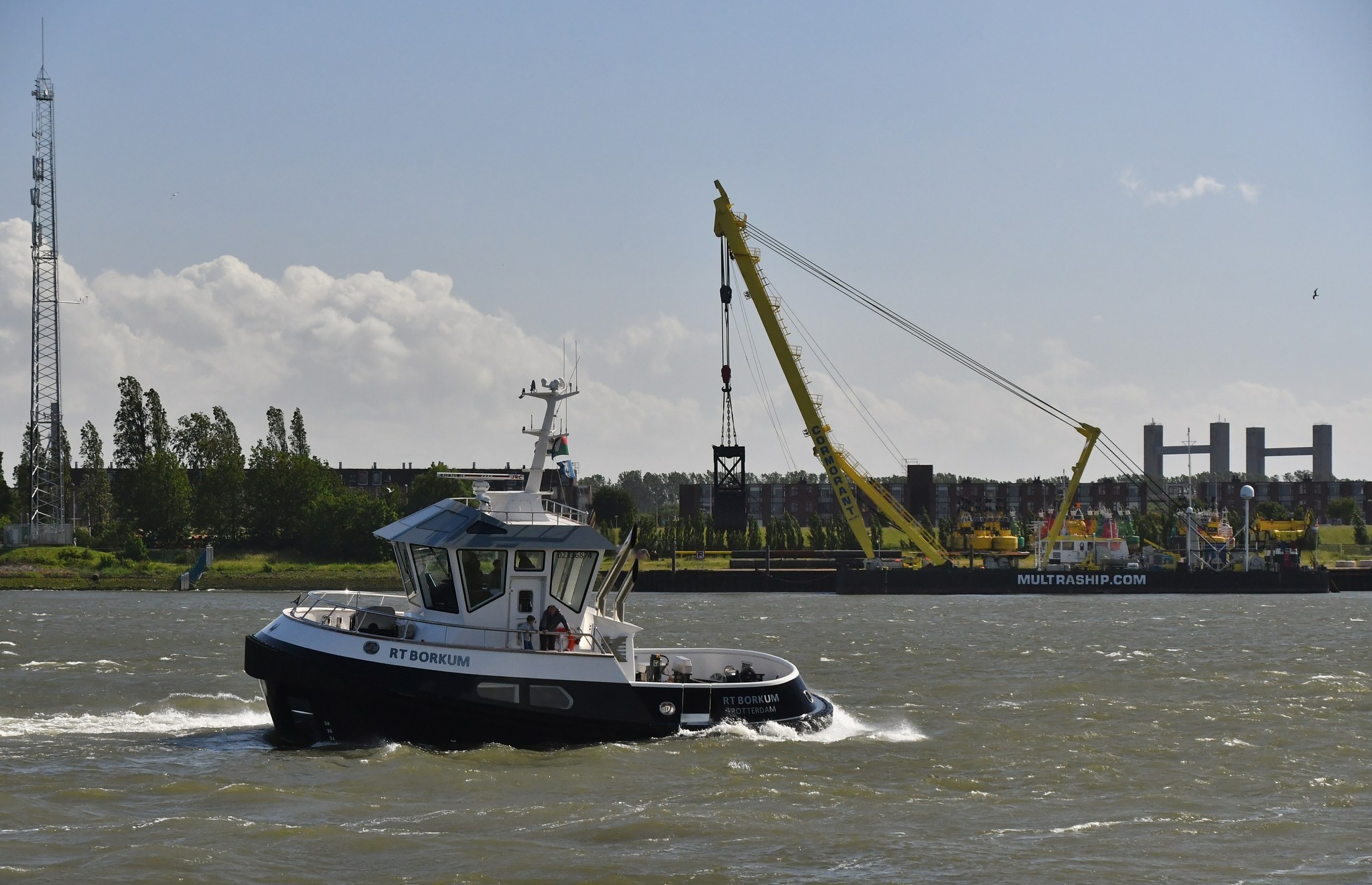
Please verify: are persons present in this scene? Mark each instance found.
[539,605,573,651]
[516,551,533,569]
[487,559,502,589]
[517,615,539,649]
[463,553,484,590]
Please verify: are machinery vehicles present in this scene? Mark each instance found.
[709,176,1101,571]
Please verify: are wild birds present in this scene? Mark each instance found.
[1312,287,1319,300]
[169,190,181,201]
[385,486,395,493]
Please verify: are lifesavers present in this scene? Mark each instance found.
[1175,565,1182,574]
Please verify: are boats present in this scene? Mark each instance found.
[1172,501,1311,572]
[1033,468,1141,569]
[243,330,836,752]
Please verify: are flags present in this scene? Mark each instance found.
[551,436,569,459]
[557,460,576,478]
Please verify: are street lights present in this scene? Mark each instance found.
[1186,506,1194,566]
[1239,485,1254,572]
